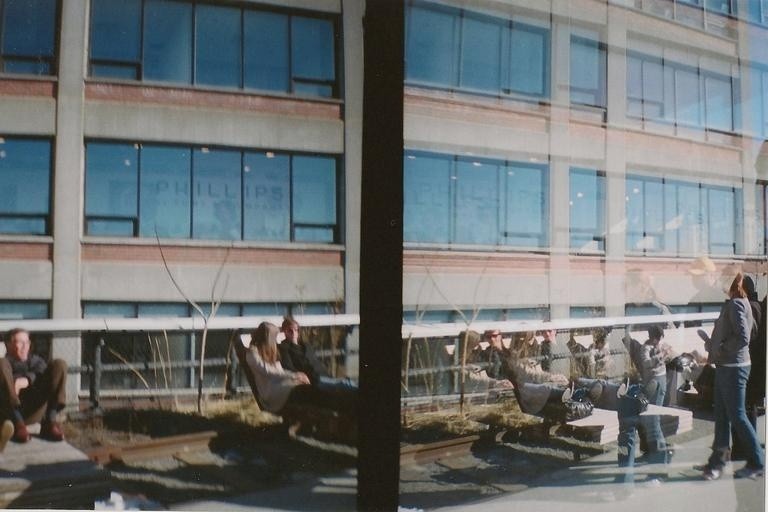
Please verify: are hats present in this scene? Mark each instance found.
[684,256,716,277]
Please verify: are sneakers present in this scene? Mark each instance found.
[697,466,765,480]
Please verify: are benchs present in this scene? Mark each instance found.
[235,331,349,472]
[447,335,598,419]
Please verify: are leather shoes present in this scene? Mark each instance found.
[13,419,31,442]
[40,418,65,442]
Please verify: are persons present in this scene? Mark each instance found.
[246,322,358,419]
[485,322,683,485]
[213,198,244,239]
[0,329,70,443]
[280,315,357,390]
[698,263,764,479]
[477,255,765,503]
